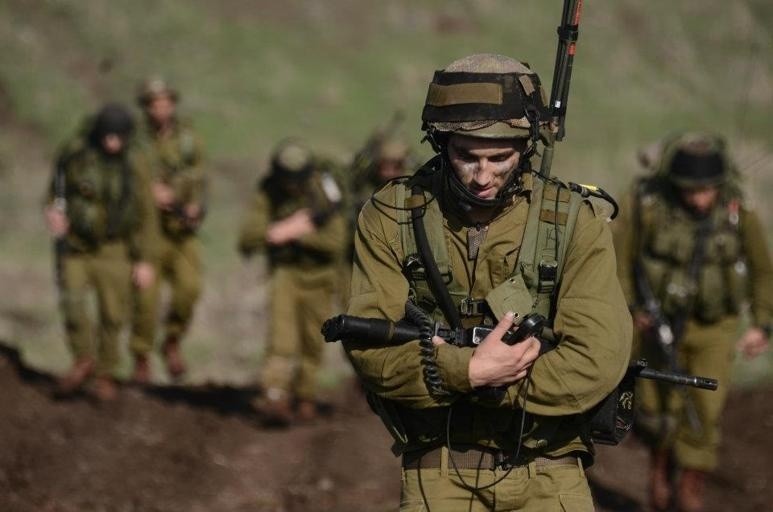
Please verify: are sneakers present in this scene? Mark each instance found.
[252,388,316,425]
[56,342,186,402]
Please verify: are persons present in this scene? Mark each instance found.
[236,138,348,422]
[129,77,208,386]
[344,51,635,511]
[603,128,773,511]
[43,103,160,402]
[342,122,411,317]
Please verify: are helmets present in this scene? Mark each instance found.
[428,54,548,139]
[660,133,730,188]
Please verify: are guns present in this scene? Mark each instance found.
[628,255,706,431]
[321,314,717,391]
